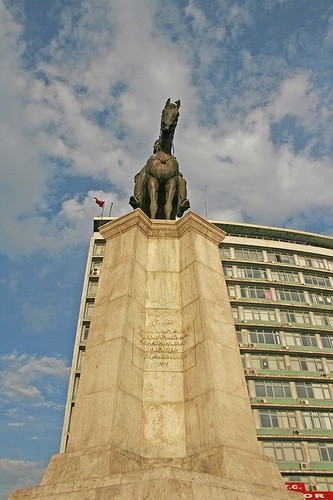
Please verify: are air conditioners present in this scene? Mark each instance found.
[300,400,308,404]
[92,271,100,275]
[300,463,310,470]
[244,369,250,376]
[250,369,257,376]
[259,399,266,403]
[282,345,290,350]
[312,485,319,492]
[224,256,332,328]
[249,343,257,348]
[293,430,302,436]
[322,373,330,378]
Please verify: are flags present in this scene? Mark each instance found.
[94,197,104,207]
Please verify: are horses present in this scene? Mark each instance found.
[143,98,181,220]
